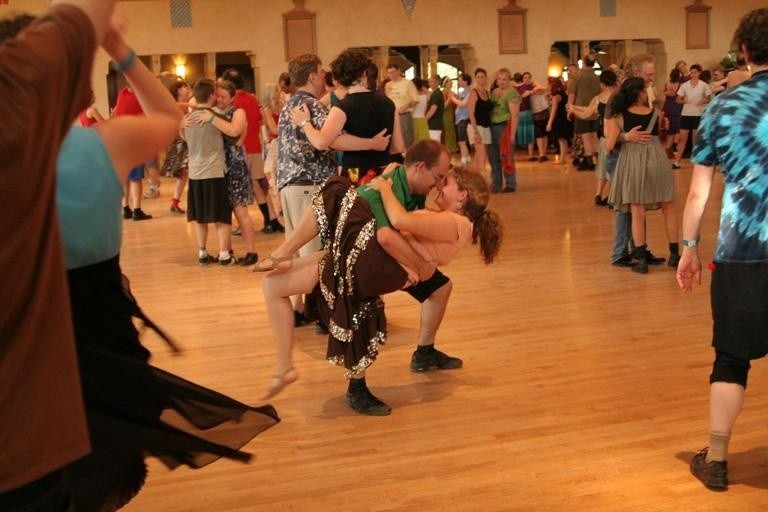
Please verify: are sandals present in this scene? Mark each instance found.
[255,366,300,401]
[248,252,294,274]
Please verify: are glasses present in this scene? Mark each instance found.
[426,165,443,184]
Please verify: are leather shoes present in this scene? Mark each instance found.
[170,206,186,214]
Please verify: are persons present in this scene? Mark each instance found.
[0,0,766,512]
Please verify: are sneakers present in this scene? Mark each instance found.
[410,350,464,373]
[538,154,549,164]
[611,253,639,267]
[261,220,287,235]
[528,156,538,162]
[214,248,240,266]
[689,451,730,493]
[133,210,152,221]
[571,153,598,172]
[631,259,649,274]
[630,246,665,265]
[231,228,242,236]
[237,251,259,266]
[124,210,133,219]
[670,163,681,169]
[195,254,214,265]
[666,253,682,267]
[345,384,392,416]
[594,193,610,206]
